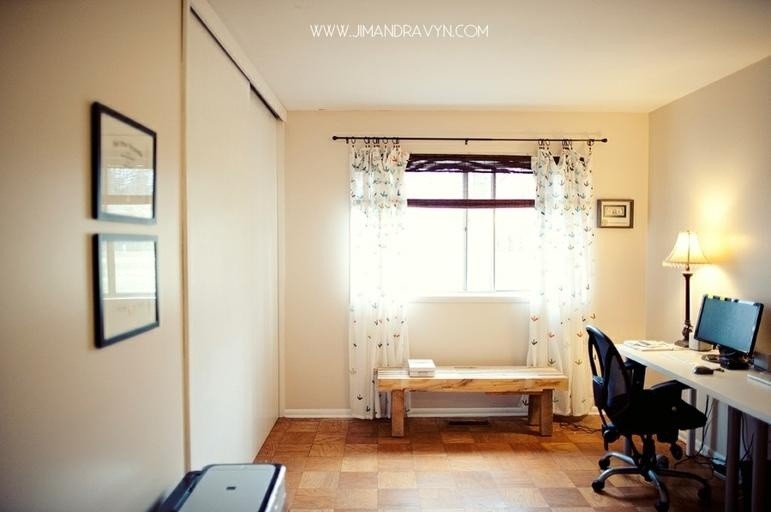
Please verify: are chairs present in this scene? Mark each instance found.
[585,324,710,512]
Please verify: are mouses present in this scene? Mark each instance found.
[693,366,713,375]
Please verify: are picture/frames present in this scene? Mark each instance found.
[92,232,161,350]
[597,198,635,229]
[92,101,159,226]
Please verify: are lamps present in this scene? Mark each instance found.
[661,230,712,348]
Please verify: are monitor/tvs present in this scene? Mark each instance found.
[693,294,764,370]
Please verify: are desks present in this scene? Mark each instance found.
[612,337,770,512]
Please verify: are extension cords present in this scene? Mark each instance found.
[712,464,742,485]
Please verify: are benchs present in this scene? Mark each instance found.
[371,364,569,440]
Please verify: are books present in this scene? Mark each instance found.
[623,338,674,352]
[746,371,771,387]
[408,366,436,378]
[407,358,436,372]
